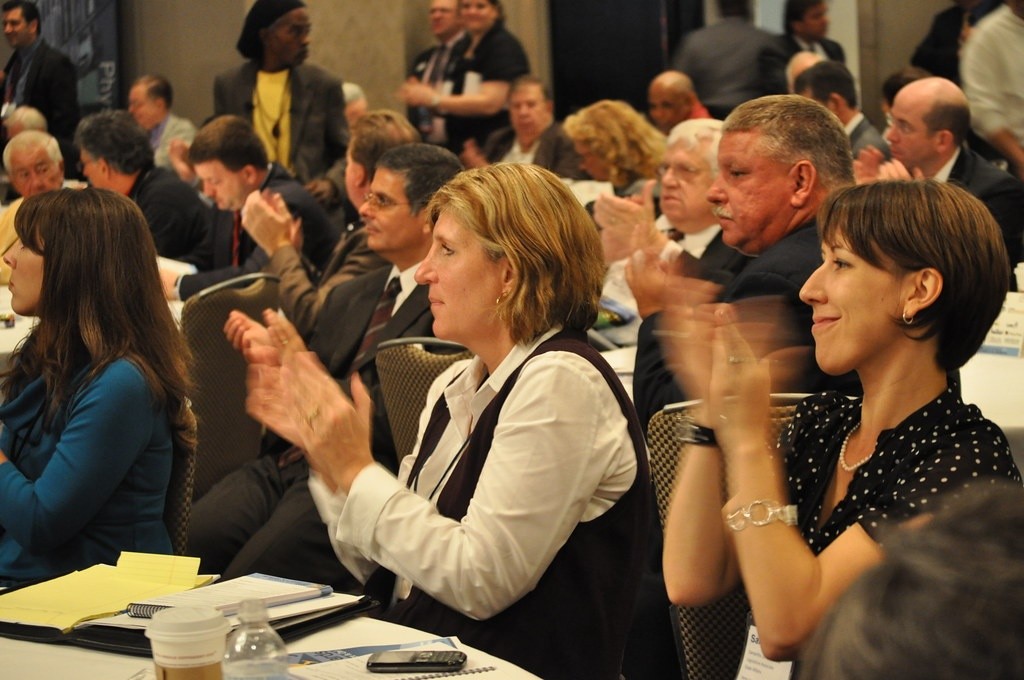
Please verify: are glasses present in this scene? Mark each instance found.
[656,164,717,180]
[887,113,925,135]
[362,190,417,208]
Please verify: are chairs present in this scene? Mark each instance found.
[167,274,859,679]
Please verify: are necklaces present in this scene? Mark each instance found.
[839,417,875,471]
[723,494,802,532]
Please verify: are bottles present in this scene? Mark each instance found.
[221,598,289,680]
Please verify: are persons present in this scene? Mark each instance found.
[214,1,349,157]
[0,1,1024,351]
[226,166,670,680]
[125,74,203,178]
[592,118,739,323]
[632,94,856,437]
[478,76,585,195]
[0,130,74,285]
[0,188,194,600]
[75,109,212,270]
[193,146,484,579]
[800,478,1024,680]
[662,181,1024,680]
[152,115,339,299]
[241,109,430,345]
[558,98,668,238]
[0,0,80,144]
[842,77,1024,269]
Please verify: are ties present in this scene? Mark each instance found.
[430,44,447,89]
[277,278,403,469]
[232,209,241,268]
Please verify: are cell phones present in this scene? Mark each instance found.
[367,651,467,673]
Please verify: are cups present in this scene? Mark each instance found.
[144,604,234,680]
[1014,262,1024,293]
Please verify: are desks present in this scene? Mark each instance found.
[1,585,546,680]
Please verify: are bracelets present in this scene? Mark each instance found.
[432,95,448,115]
[678,418,721,450]
